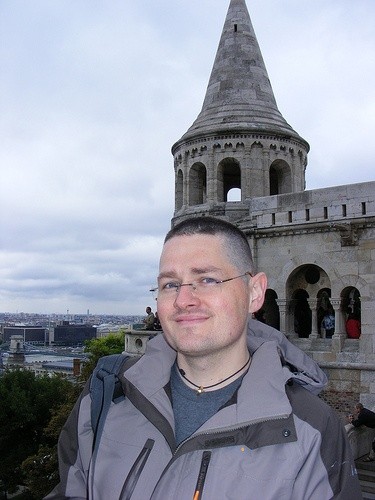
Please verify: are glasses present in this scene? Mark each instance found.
[150,272,252,301]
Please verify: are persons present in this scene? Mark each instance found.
[252,309,277,328]
[321,308,335,338]
[153,311,161,330]
[57,216,361,500]
[346,307,360,338]
[142,307,155,330]
[348,403,375,462]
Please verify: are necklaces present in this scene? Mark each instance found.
[173,350,251,394]
